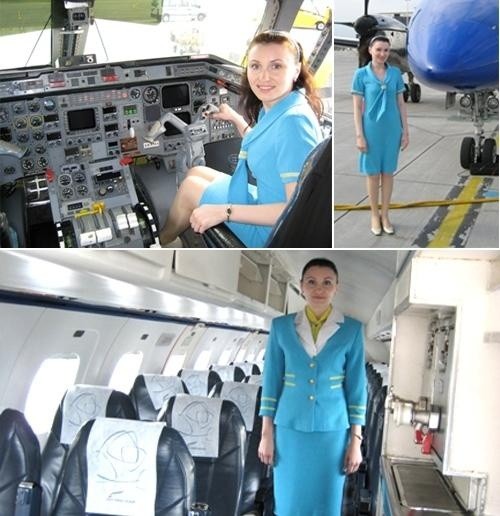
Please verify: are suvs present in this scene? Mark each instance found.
[152,0,209,24]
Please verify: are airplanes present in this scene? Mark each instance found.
[334,1,499,176]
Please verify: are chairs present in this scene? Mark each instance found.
[179,135,333,247]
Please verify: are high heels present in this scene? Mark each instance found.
[381,216,396,234]
[370,216,383,236]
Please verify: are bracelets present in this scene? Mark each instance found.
[226,202,233,223]
[351,432,364,442]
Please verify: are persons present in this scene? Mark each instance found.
[257,257,368,516]
[352,35,410,235]
[148,29,326,248]
